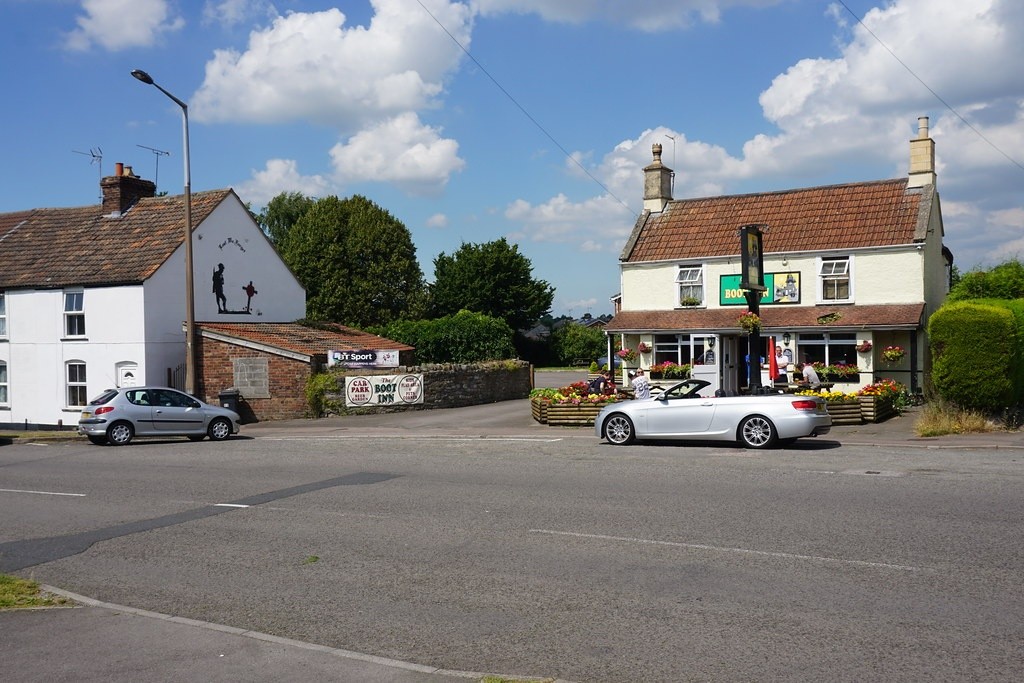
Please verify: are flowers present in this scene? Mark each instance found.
[792,389,860,403]
[856,379,899,398]
[793,361,860,377]
[617,348,638,363]
[736,310,762,337]
[882,344,908,364]
[649,360,691,380]
[529,381,617,406]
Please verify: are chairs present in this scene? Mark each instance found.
[714,389,726,398]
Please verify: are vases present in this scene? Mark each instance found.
[792,373,859,382]
[825,401,865,423]
[855,394,896,422]
[530,398,611,425]
[650,371,687,379]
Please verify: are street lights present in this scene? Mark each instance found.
[131,68,196,397]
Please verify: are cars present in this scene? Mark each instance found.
[598,355,621,369]
[77,385,241,445]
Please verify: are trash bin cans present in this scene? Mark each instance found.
[217,389,240,413]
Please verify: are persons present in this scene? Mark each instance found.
[592,370,618,397]
[745,353,764,385]
[628,368,651,400]
[796,363,821,394]
[775,346,790,388]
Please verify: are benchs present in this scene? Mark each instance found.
[751,385,771,395]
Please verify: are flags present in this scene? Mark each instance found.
[770,340,781,380]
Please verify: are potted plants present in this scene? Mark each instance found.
[681,297,700,306]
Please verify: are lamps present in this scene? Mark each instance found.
[707,337,715,347]
[783,332,791,344]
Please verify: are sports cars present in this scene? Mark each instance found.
[595,379,833,449]
